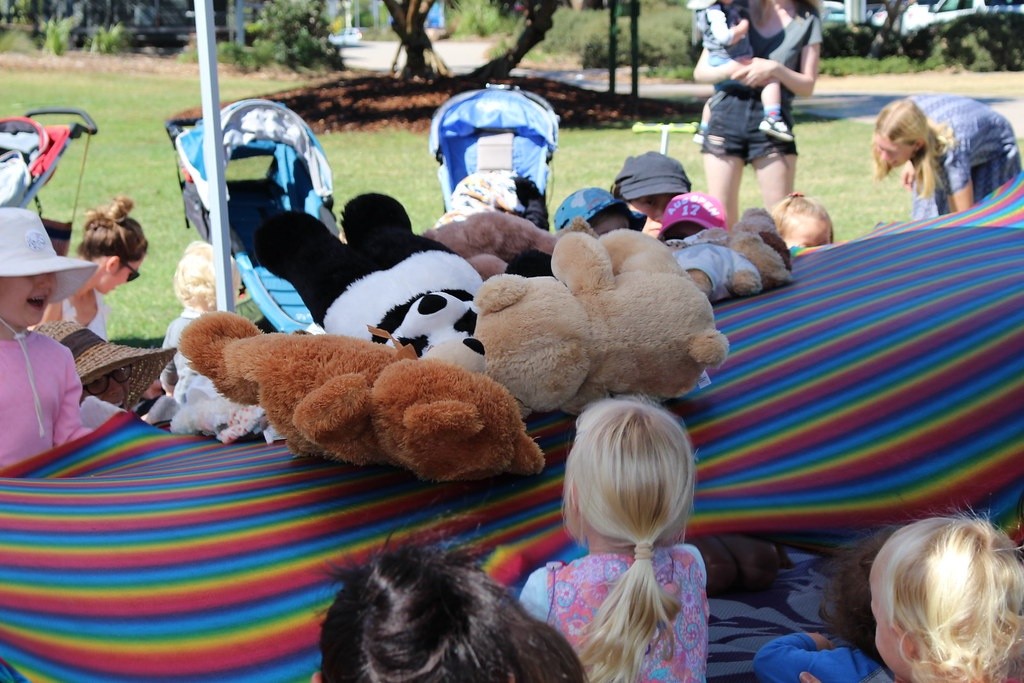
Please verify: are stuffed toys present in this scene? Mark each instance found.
[259,193,796,375]
[470,225,735,414]
[176,317,547,481]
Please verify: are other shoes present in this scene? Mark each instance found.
[758,116,794,142]
[692,126,726,155]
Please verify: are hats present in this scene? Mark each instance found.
[0,207,98,305]
[655,191,726,244]
[555,187,647,237]
[611,151,692,203]
[30,320,179,412]
[686,0,716,10]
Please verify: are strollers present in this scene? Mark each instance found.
[0,107,98,256]
[430,84,559,231]
[165,99,335,334]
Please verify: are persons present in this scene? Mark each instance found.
[611,153,693,238]
[798,516,1024,683]
[26,198,149,341]
[689,0,796,146]
[305,551,591,682]
[755,525,892,683]
[655,194,732,246]
[160,240,243,397]
[870,90,1023,220]
[34,321,179,412]
[552,187,633,236]
[0,207,100,474]
[516,396,712,683]
[773,190,835,249]
[693,0,823,231]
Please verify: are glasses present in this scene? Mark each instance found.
[81,362,132,395]
[114,255,140,282]
